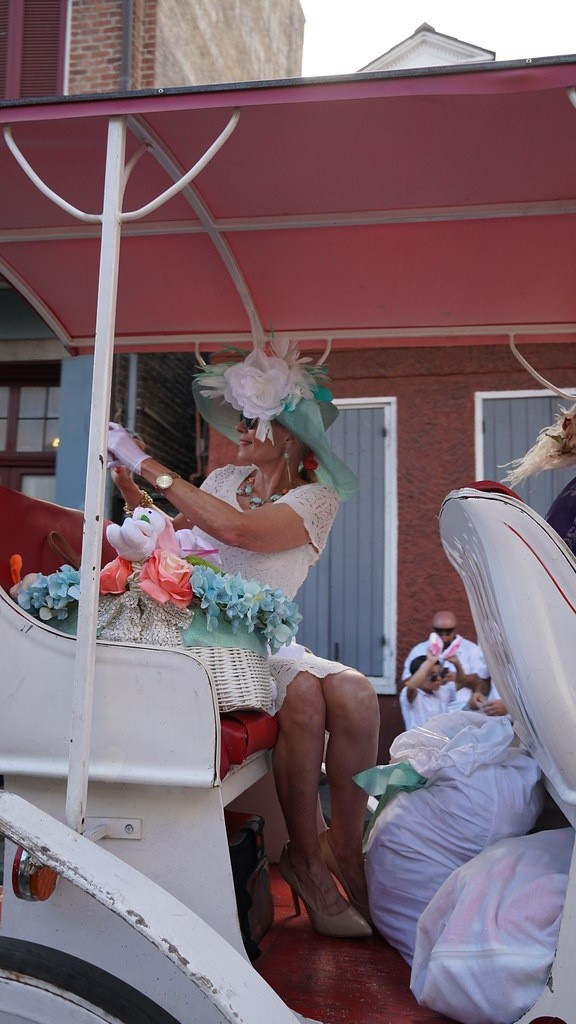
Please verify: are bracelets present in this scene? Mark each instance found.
[124,490,153,516]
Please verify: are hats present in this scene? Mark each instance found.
[193,321,358,502]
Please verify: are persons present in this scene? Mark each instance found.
[397,611,522,748]
[544,473,576,559]
[107,365,387,940]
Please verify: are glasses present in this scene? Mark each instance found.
[433,627,456,636]
[430,667,449,681]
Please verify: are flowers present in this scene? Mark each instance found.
[198,333,336,443]
[14,554,302,645]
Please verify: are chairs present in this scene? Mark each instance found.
[435,484,575,828]
[0,483,287,783]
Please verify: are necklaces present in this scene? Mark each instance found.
[235,467,306,510]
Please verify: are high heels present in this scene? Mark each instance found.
[278,828,376,938]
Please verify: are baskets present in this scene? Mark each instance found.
[97,507,302,714]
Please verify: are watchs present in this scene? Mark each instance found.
[155,471,180,495]
